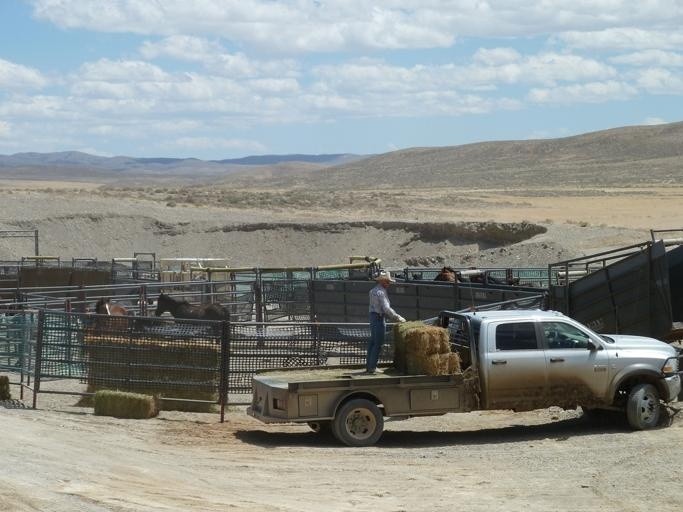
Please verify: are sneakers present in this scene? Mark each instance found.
[366,367,385,375]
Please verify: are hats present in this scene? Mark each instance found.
[373,270,397,283]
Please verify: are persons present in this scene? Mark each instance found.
[366,271,408,374]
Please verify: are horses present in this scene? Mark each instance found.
[154,292,230,336]
[95,297,129,331]
[433,267,461,283]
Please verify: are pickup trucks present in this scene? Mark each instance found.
[245,308,682,447]
